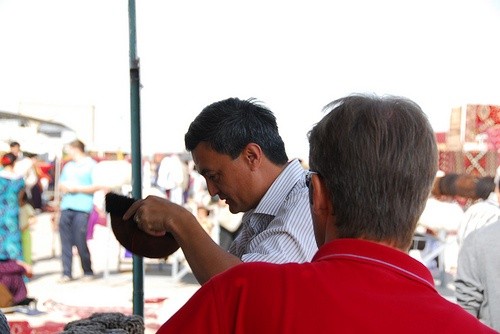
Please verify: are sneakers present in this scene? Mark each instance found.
[81,272,96,282]
[61,274,73,284]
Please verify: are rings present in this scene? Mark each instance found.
[135,215,142,224]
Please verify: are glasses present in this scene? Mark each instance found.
[305,170,315,185]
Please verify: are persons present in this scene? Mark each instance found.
[0,153,30,283]
[122,97,319,286]
[0,256,35,310]
[407,167,500,332]
[58,139,103,283]
[155,92,500,334]
[10,141,59,215]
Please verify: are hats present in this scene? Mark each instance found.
[103,190,183,259]
